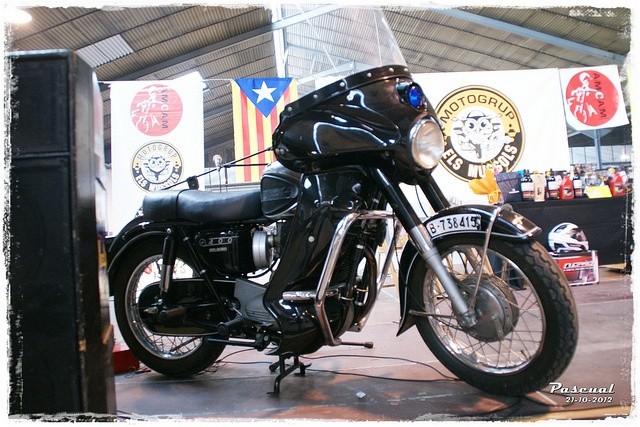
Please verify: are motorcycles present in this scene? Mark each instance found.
[106,5,581,398]
[519,163,579,198]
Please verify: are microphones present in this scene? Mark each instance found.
[211,154,224,172]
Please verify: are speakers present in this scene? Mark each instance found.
[6,45,119,413]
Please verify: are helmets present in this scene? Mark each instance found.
[548,221,589,253]
